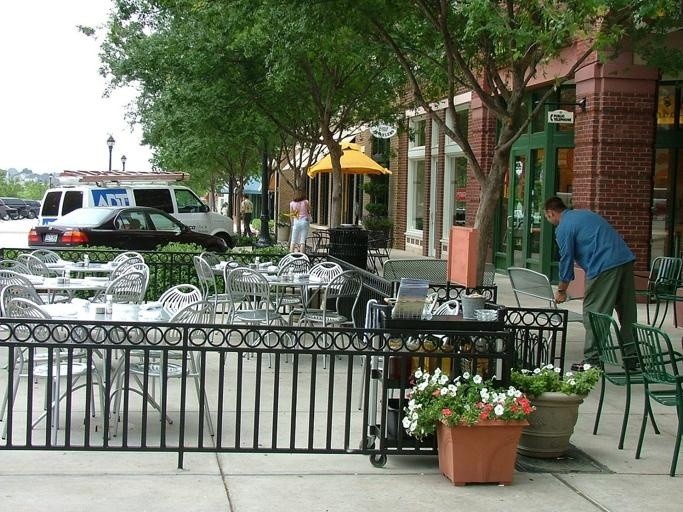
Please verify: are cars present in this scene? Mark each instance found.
[27,206,227,253]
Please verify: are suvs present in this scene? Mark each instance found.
[0,199,19,220]
[3,198,28,219]
[24,200,41,219]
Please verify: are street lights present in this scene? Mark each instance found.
[48,173,53,189]
[121,155,127,172]
[107,135,116,171]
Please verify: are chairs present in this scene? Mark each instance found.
[297,271,364,370]
[1,286,95,422]
[588,309,659,442]
[0,261,33,274]
[358,299,386,413]
[92,251,172,439]
[276,253,309,312]
[483,262,497,286]
[433,300,461,316]
[647,256,681,327]
[305,228,398,279]
[378,258,448,281]
[15,254,87,362]
[508,267,584,364]
[114,302,214,437]
[192,256,232,343]
[200,252,233,314]
[0,271,36,291]
[1,298,110,445]
[225,269,288,366]
[276,259,310,313]
[422,293,438,318]
[27,250,75,303]
[631,323,683,477]
[284,262,344,352]
[158,284,202,337]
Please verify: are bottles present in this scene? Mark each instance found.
[106,295,112,313]
[65,269,70,285]
[255,257,259,266]
[84,255,89,266]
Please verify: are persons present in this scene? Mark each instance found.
[290,190,311,253]
[240,194,254,237]
[543,197,641,372]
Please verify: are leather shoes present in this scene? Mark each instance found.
[571,358,605,372]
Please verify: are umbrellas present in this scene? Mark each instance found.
[307,143,393,178]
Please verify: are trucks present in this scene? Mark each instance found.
[38,183,234,248]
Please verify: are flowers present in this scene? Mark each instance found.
[508,354,603,396]
[401,364,534,438]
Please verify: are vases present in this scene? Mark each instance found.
[433,417,531,484]
[513,388,587,458]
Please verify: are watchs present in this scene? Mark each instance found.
[557,289,566,294]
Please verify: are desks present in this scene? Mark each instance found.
[18,299,187,422]
[32,255,116,279]
[0,273,112,305]
[210,259,280,317]
[232,270,349,353]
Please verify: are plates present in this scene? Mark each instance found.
[473,309,497,321]
